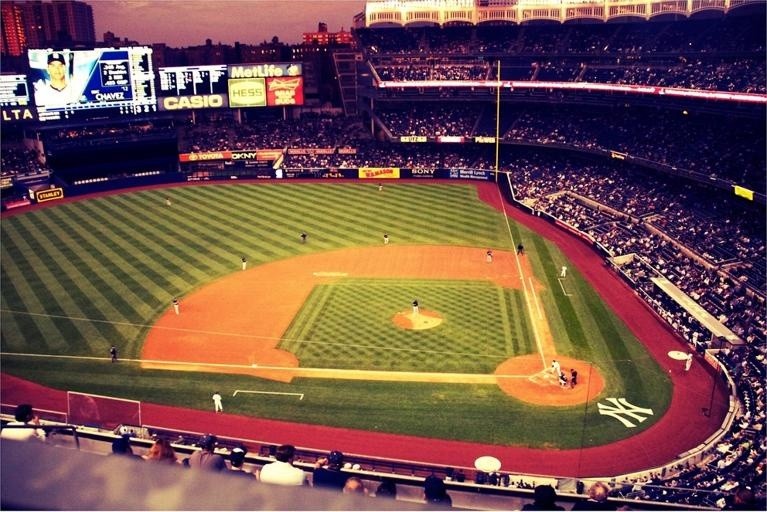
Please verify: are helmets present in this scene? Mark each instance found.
[200,435,217,445]
[328,450,344,463]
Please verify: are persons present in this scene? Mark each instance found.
[0,39,767,511]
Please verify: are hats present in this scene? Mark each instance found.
[230,446,247,460]
